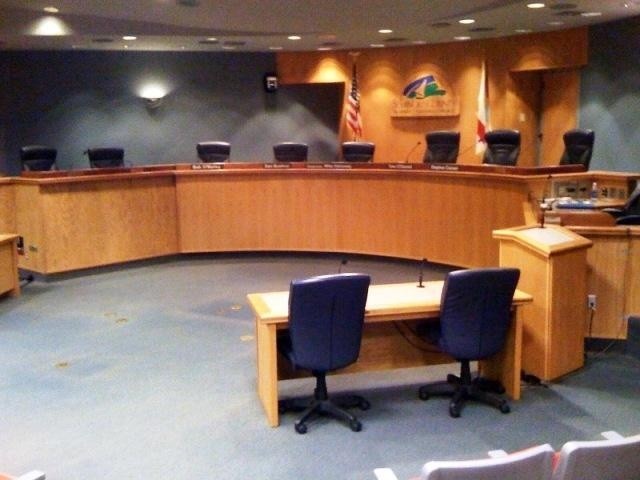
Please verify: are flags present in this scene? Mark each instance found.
[475,62,493,154]
[344,65,365,138]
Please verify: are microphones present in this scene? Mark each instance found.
[451,138,482,163]
[69,151,89,170]
[541,175,552,201]
[514,133,542,165]
[406,140,422,162]
[338,259,348,273]
[417,258,427,288]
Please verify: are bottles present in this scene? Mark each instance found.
[590,183,598,203]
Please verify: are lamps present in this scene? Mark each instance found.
[143,98,161,109]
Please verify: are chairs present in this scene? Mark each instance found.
[371,430,640,480]
[0,469,45,480]
